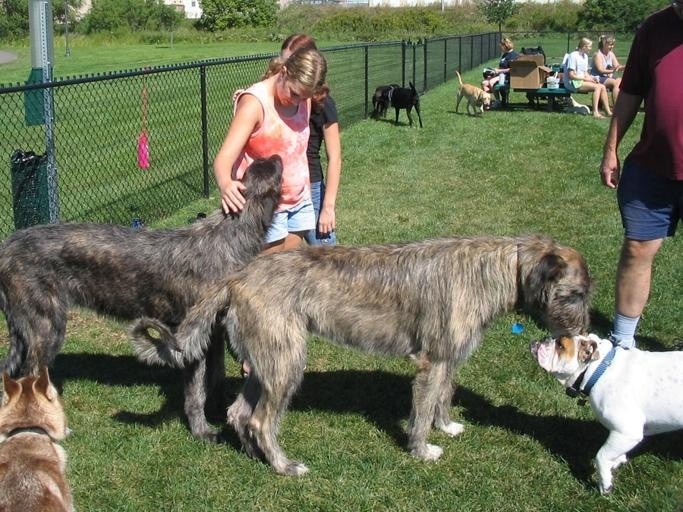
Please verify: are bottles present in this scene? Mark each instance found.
[138,132,150,167]
[499,71,505,86]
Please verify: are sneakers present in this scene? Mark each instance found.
[490,102,503,108]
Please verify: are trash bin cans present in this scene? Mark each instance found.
[10,149,50,231]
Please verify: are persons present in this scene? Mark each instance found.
[600,2,683,348]
[563,38,612,118]
[269,33,340,246]
[213,49,328,255]
[481,38,518,110]
[590,35,625,107]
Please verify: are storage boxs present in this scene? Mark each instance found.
[505,52,554,92]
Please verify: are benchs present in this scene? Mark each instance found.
[492,83,511,105]
[521,83,575,113]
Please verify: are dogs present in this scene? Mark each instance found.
[0,153,284,445]
[0,365,75,512]
[529,332,683,500]
[379,81,423,128]
[122,232,594,478]
[372,83,400,122]
[455,70,495,117]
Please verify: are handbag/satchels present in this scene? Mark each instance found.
[547,71,560,90]
